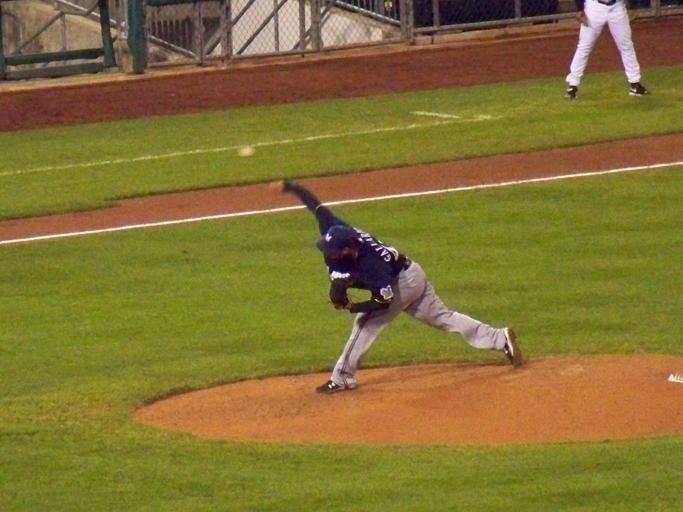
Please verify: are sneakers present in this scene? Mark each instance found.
[503,327,523,370]
[629,82,647,97]
[315,380,357,395]
[563,86,578,100]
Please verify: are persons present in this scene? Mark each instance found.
[564,0,647,100]
[268,178,522,394]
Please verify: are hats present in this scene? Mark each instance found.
[315,224,361,256]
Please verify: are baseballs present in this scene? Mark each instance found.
[237,145,255,160]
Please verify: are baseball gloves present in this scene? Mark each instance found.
[328,276,354,307]
